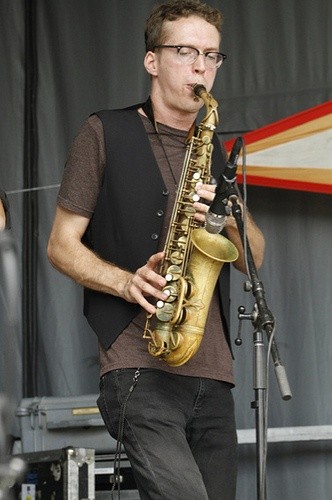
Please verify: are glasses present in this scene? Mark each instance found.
[153,45,227,69]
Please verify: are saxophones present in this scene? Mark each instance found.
[142,82,239,368]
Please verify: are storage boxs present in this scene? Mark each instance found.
[0,445,140,500]
[14,390,128,456]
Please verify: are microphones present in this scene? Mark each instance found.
[202,136,242,235]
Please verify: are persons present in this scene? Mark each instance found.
[46,1,266,500]
